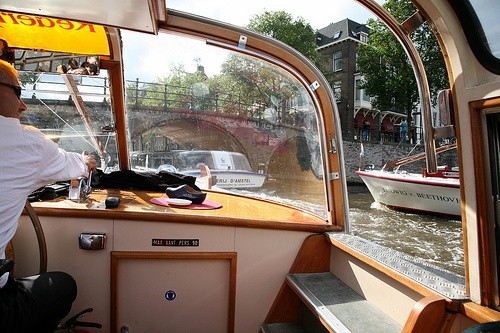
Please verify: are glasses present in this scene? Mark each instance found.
[0,82,22,100]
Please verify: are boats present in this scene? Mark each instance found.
[355,125,462,221]
[130,149,268,190]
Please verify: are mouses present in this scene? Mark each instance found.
[105,197,120,207]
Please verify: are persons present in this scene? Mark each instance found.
[36,58,95,76]
[393,118,417,145]
[0,61,97,333]
